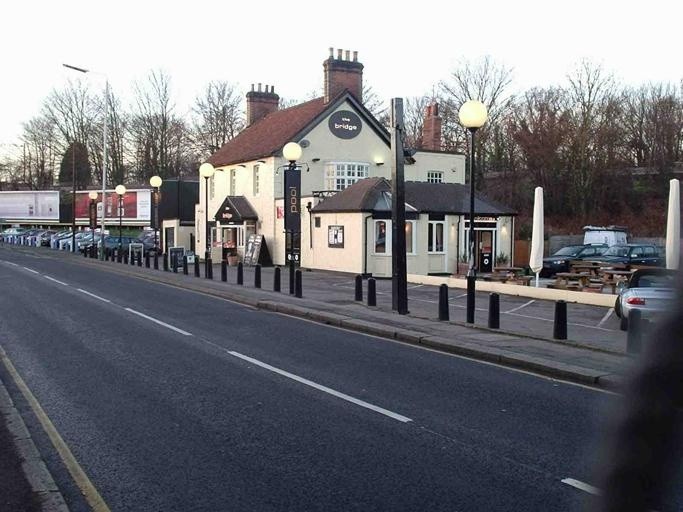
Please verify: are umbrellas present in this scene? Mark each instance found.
[527,186,545,287]
[662,177,681,270]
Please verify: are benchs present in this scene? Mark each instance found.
[483,275,534,286]
[550,261,632,293]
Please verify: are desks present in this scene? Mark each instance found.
[483,265,534,275]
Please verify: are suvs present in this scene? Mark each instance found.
[585,242,666,282]
[540,242,610,279]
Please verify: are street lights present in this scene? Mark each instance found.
[456,98,487,278]
[115,184,124,252]
[282,142,303,298]
[197,162,214,280]
[149,175,163,269]
[87,189,98,257]
[59,62,110,262]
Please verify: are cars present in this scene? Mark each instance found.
[0,227,162,260]
[614,266,682,331]
